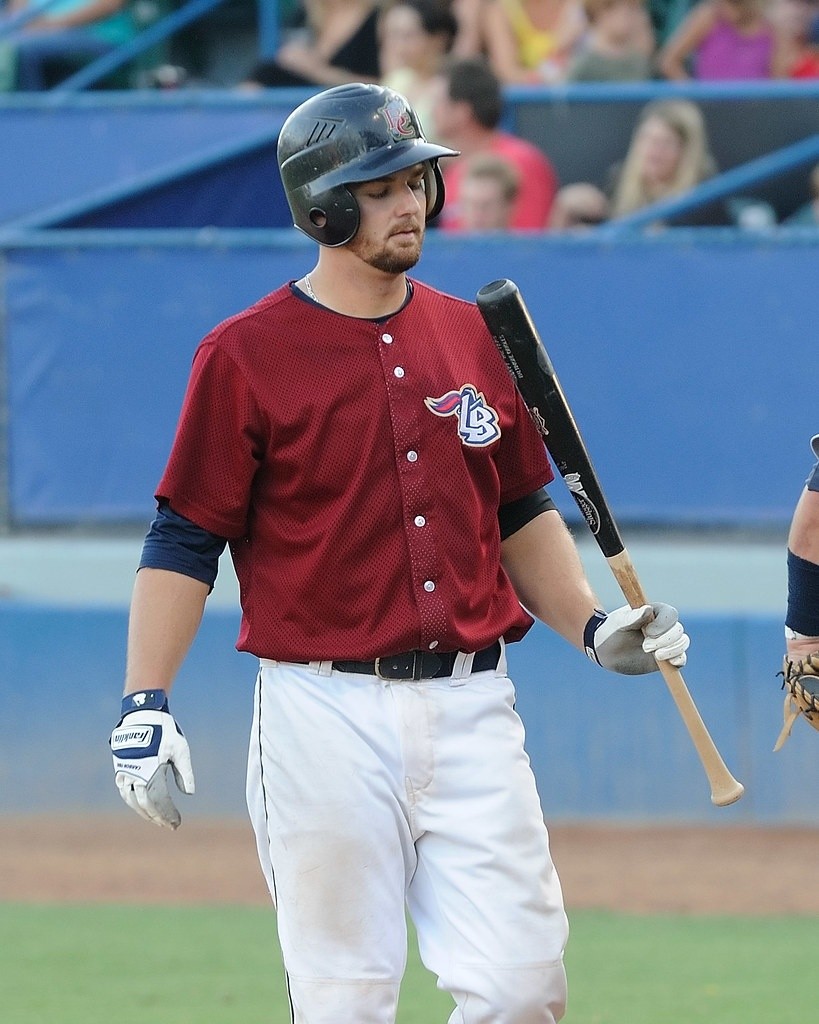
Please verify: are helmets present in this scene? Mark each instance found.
[277,83,461,248]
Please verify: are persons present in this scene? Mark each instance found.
[106,80,691,1023]
[242,0,819,231]
[782,428,819,738]
[11,1,157,98]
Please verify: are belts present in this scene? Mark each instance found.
[289,649,501,680]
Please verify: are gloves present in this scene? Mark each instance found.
[583,603,690,676]
[108,689,195,831]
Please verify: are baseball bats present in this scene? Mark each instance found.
[474,273,749,810]
